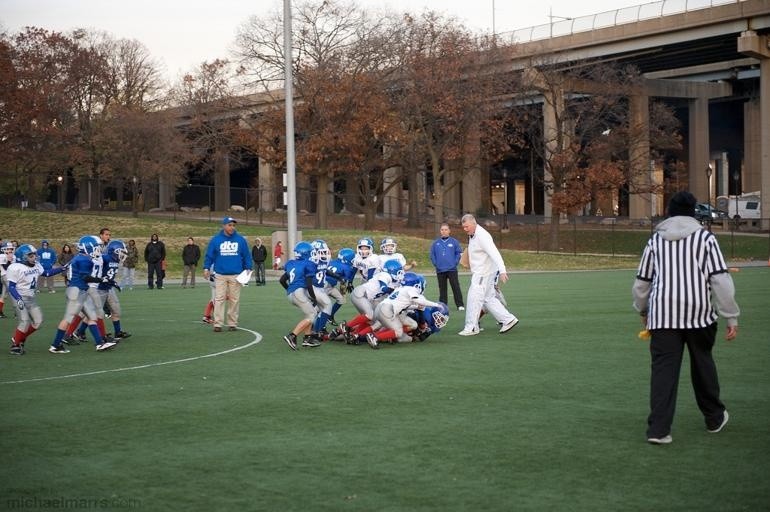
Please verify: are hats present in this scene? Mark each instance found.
[223,216,237,224]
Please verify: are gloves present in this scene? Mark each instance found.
[63,261,72,271]
[112,281,121,291]
[312,299,318,307]
[17,299,25,310]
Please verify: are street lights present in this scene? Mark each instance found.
[705,163,713,229]
[132,174,139,217]
[547,13,573,41]
[501,167,508,229]
[732,169,740,227]
[56,173,63,210]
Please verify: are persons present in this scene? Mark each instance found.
[431,222,465,311]
[273,241,284,270]
[180,237,201,288]
[633,190,740,445]
[457,213,519,336]
[0,228,138,356]
[252,238,267,286]
[203,216,253,332]
[144,234,166,289]
[203,272,229,324]
[279,239,448,351]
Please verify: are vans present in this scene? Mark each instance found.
[717,193,762,227]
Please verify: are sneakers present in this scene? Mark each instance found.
[704,411,729,433]
[0,311,132,355]
[648,436,673,445]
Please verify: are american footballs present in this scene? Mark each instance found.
[418,322,428,332]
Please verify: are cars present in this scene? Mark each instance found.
[689,203,729,231]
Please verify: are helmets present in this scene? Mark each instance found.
[0,235,129,263]
[293,237,427,296]
